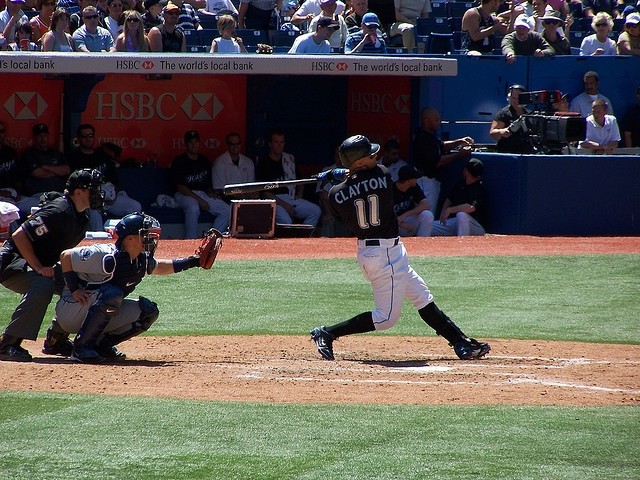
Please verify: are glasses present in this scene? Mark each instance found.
[596,23,608,27]
[229,141,240,145]
[545,19,559,24]
[80,134,94,137]
[85,16,99,20]
[364,24,379,29]
[627,24,638,28]
[126,18,141,22]
[111,2,122,7]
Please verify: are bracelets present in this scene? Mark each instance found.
[61,269,81,295]
[172,257,190,273]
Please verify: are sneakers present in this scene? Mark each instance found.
[0,341,31,362]
[455,339,491,361]
[73,344,105,362]
[310,325,334,360]
[42,337,71,356]
[97,344,126,359]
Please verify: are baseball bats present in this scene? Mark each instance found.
[223,178,318,195]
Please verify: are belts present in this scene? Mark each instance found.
[361,237,401,248]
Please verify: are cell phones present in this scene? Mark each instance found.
[20,40,30,51]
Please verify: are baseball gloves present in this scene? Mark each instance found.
[195,230,223,268]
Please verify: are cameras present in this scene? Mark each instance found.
[516,90,587,152]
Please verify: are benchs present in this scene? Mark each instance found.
[150,146,339,236]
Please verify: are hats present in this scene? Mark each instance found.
[339,135,380,165]
[32,124,49,135]
[514,14,532,28]
[186,132,199,143]
[10,0,25,3]
[538,10,564,22]
[467,157,485,175]
[317,17,340,30]
[399,165,424,180]
[163,3,180,14]
[626,13,640,24]
[115,212,161,237]
[320,0,338,4]
[362,13,380,27]
[66,169,106,188]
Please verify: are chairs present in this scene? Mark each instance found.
[411,3,636,55]
[181,6,410,53]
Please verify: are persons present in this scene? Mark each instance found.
[72,6,114,52]
[580,0,620,19]
[375,138,408,182]
[580,12,617,55]
[345,12,388,53]
[185,0,207,15]
[390,0,430,53]
[311,134,490,361]
[0,121,41,234]
[148,4,186,51]
[578,98,621,154]
[287,16,335,53]
[544,0,567,16]
[237,0,282,29]
[307,0,347,47]
[289,0,346,25]
[211,132,260,199]
[57,0,81,13]
[204,0,240,14]
[345,0,383,30]
[62,124,142,231]
[550,92,570,113]
[22,124,72,193]
[412,107,475,180]
[617,12,640,55]
[98,0,109,22]
[175,130,230,238]
[0,0,29,42]
[162,0,203,30]
[433,157,489,237]
[255,130,322,238]
[281,0,301,16]
[392,163,435,236]
[528,0,575,43]
[570,71,613,116]
[0,168,104,361]
[489,85,532,154]
[209,15,246,52]
[8,22,40,51]
[500,14,556,64]
[116,11,150,51]
[496,0,533,17]
[140,1,164,34]
[623,84,639,146]
[44,9,75,52]
[538,9,571,55]
[55,211,200,361]
[103,0,124,40]
[29,0,55,43]
[459,0,515,55]
[70,0,107,31]
